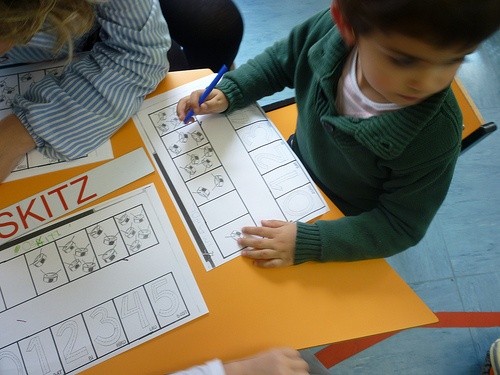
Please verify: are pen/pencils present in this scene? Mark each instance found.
[182,65,228,125]
[0,208,95,252]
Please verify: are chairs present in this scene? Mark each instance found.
[260,72,497,156]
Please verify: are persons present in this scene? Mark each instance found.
[0,0,245,183]
[176,0,500,268]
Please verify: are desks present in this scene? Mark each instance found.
[0,67,439,375]
